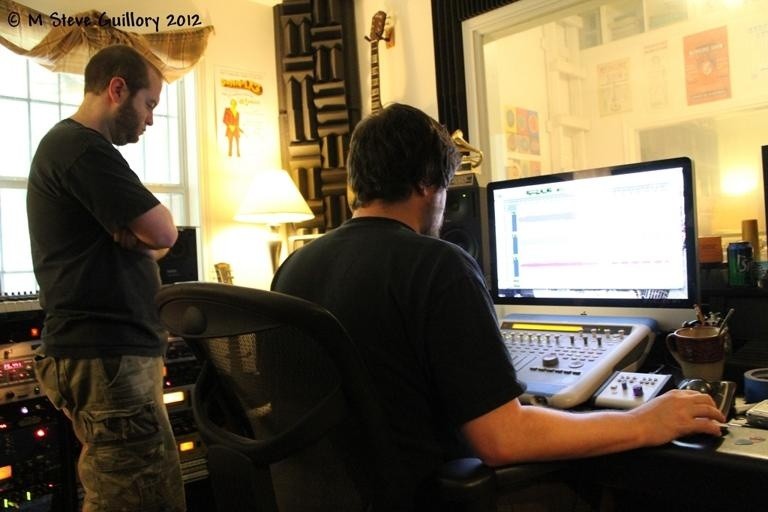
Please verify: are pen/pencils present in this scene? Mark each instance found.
[693,304,706,326]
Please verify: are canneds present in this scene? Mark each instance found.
[727,241,754,288]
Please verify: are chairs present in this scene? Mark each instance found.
[153,281,603,510]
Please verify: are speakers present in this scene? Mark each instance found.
[154,226,198,284]
[437,188,484,275]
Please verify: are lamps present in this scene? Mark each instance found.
[229,169,315,280]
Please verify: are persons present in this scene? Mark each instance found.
[26,44,189,511]
[242,101,732,512]
[223,98,241,156]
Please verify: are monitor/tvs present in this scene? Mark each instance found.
[487,157,700,336]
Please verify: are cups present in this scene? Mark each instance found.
[666,327,726,384]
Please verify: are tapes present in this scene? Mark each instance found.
[744,368,768,404]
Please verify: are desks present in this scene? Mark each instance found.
[587,372,763,473]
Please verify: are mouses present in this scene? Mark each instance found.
[695,415,729,431]
